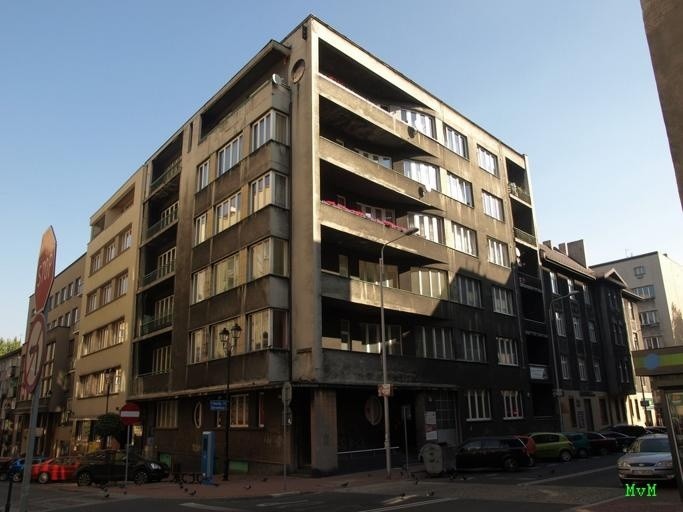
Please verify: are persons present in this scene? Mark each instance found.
[142,437,157,459]
[527,433,537,467]
[111,436,121,450]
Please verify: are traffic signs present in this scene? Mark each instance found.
[211,401,228,410]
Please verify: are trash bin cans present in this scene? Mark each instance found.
[420,443,457,477]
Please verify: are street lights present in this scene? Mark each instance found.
[548,291,581,431]
[103,367,116,414]
[635,322,662,426]
[219,324,243,482]
[374,228,421,477]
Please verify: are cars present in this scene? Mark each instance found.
[0,447,170,488]
[447,423,682,490]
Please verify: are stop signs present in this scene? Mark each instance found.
[119,403,141,424]
[35,227,57,312]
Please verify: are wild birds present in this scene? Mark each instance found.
[341,481,349,488]
[188,490,197,497]
[243,480,252,491]
[398,492,405,499]
[104,493,110,499]
